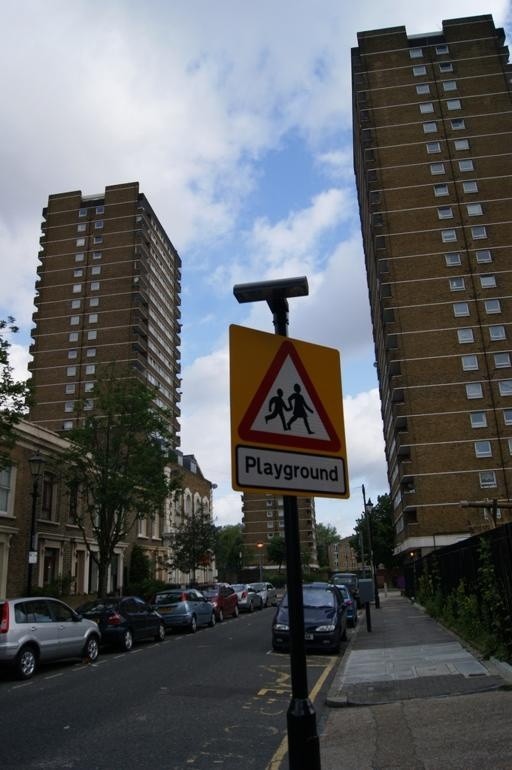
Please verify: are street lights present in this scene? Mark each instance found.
[366,496,380,609]
[257,542,264,582]
[27,447,48,594]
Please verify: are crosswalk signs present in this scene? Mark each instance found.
[228,324,350,499]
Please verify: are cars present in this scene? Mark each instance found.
[273,573,359,652]
[76,584,261,653]
[1,596,103,680]
[249,582,277,611]
